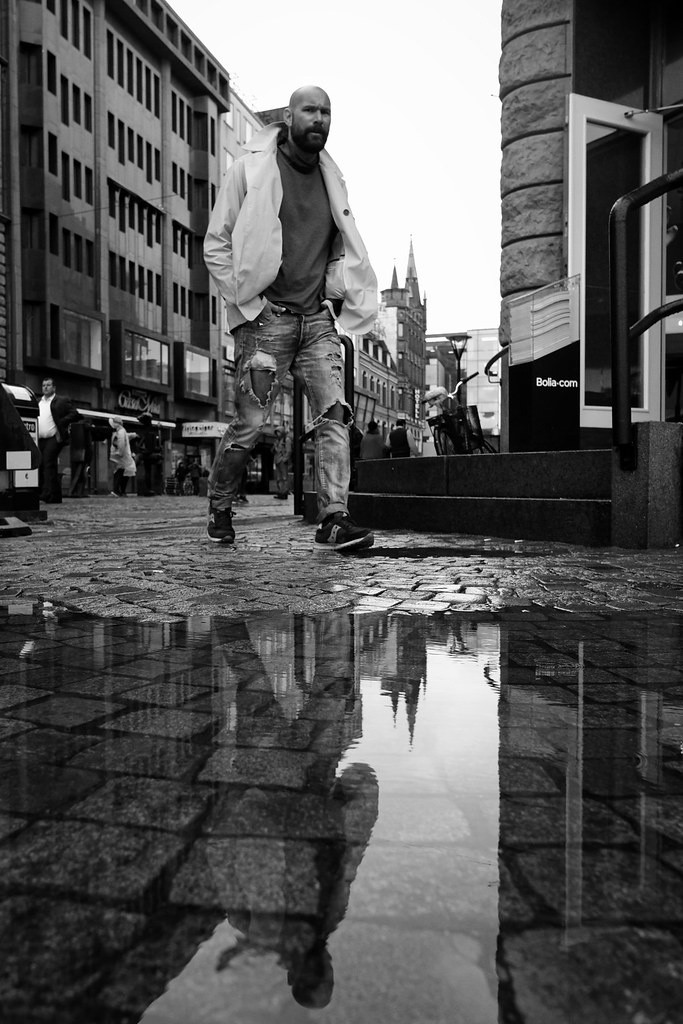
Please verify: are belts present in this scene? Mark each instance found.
[280,308,304,316]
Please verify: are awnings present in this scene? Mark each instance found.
[77,409,175,428]
[180,422,276,444]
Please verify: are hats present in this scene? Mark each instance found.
[365,420,379,434]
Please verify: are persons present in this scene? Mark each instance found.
[210,614,382,1010]
[37,380,79,503]
[202,86,380,550]
[233,467,248,504]
[349,418,420,460]
[271,426,291,500]
[72,412,202,499]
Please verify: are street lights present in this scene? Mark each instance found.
[444,331,472,454]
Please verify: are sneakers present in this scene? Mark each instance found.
[205,500,239,544]
[313,511,376,550]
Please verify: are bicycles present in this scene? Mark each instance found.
[419,372,498,457]
